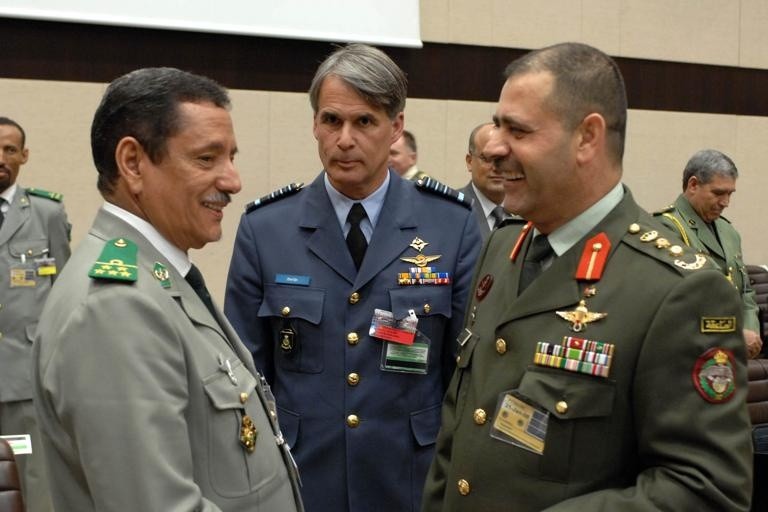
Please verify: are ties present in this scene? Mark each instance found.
[346,204,368,271]
[185,264,221,324]
[493,206,506,228]
[518,234,554,295]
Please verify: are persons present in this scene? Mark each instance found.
[30,66,306,512]
[388,130,425,183]
[0,114,73,512]
[222,41,483,511]
[650,147,763,359]
[453,122,516,242]
[419,40,757,511]
